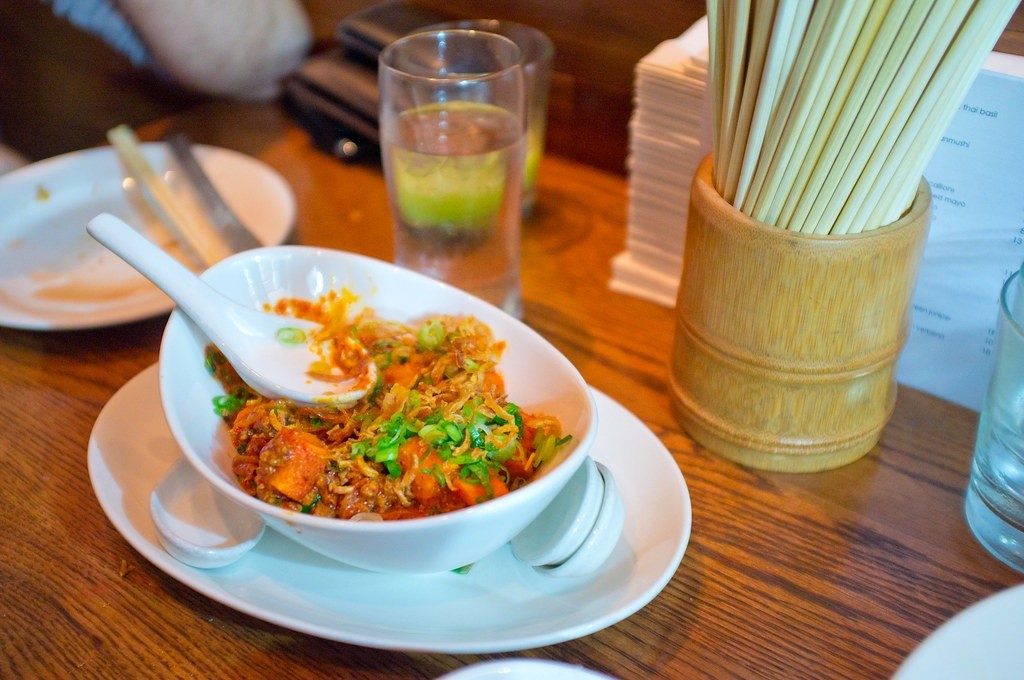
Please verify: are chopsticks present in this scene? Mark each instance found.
[705,0,1024,237]
[107,123,218,269]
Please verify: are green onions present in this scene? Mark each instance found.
[203,319,575,514]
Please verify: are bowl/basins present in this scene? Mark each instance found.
[159,242,600,573]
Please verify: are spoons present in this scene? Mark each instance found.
[532,462,624,581]
[147,458,266,569]
[84,211,376,409]
[510,454,604,568]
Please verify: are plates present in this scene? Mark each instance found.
[0,140,296,330]
[891,583,1024,680]
[439,658,614,680]
[89,360,693,652]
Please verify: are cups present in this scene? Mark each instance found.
[379,30,522,316]
[963,262,1024,570]
[400,19,553,216]
[666,146,931,473]
